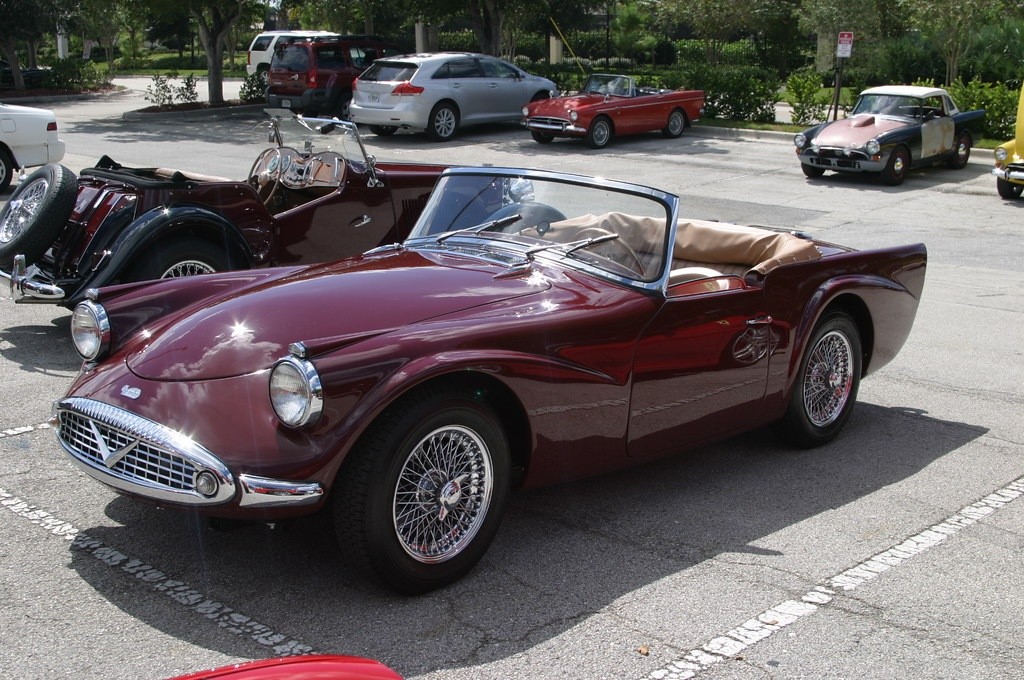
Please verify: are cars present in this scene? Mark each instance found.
[0,101,66,193]
[989,79,1024,199]
[793,85,986,186]
[0,58,52,86]
[345,50,559,142]
[0,109,568,317]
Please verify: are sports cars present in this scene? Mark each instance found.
[520,73,706,149]
[51,161,928,597]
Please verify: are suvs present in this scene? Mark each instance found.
[265,35,410,128]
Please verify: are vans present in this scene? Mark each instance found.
[247,30,366,86]
